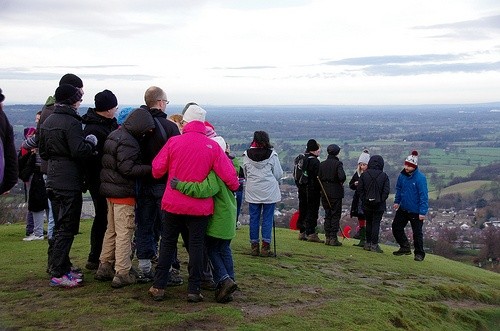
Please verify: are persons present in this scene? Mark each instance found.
[19,72,248,301]
[240,129,283,257]
[318,144,347,246]
[294,138,325,244]
[0,87,17,199]
[358,155,389,254]
[347,149,374,248]
[389,149,429,260]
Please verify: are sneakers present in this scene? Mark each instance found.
[49,262,236,303]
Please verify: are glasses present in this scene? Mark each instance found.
[179,120,184,126]
[157,99,170,104]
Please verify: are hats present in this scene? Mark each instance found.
[327,144,340,155]
[55,84,82,105]
[183,105,206,122]
[307,139,320,152]
[405,151,418,168]
[60,74,83,88]
[358,150,371,165]
[95,90,118,112]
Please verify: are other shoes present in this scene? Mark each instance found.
[260,248,276,256]
[370,243,383,253]
[22,233,44,241]
[306,233,325,243]
[325,237,342,246]
[252,249,259,255]
[353,240,365,246]
[298,234,307,240]
[392,246,411,256]
[416,254,424,261]
[365,242,371,251]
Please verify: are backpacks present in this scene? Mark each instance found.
[364,171,382,206]
[294,154,315,188]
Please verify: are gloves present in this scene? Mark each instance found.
[85,135,98,146]
[170,179,179,189]
